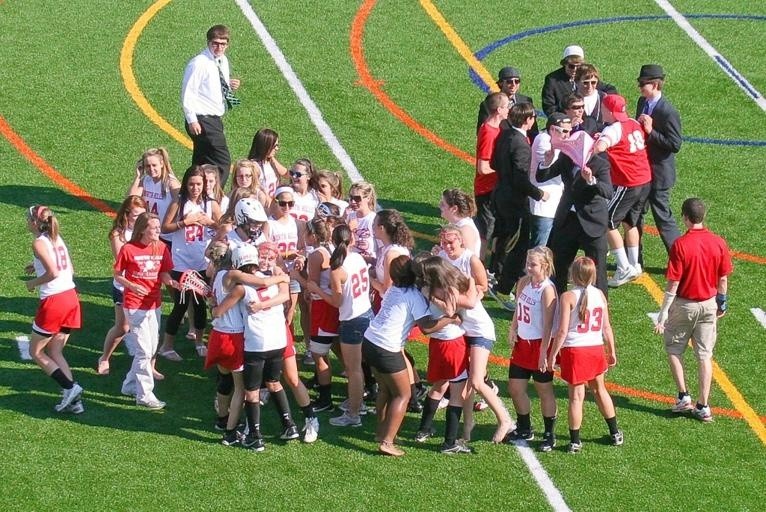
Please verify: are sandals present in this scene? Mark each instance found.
[374,434,407,456]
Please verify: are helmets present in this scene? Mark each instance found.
[234,198,268,226]
[231,241,259,268]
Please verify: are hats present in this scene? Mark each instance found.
[496,67,521,85]
[602,94,630,124]
[636,64,666,82]
[560,45,584,66]
[539,111,572,132]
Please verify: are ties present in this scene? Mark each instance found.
[215,58,241,112]
[644,99,649,116]
[569,78,576,91]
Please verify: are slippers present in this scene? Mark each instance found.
[158,347,183,362]
[184,331,209,358]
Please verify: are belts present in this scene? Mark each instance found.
[196,114,223,120]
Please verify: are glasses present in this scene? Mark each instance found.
[288,169,308,178]
[552,124,571,134]
[570,104,586,110]
[637,81,653,87]
[564,64,582,70]
[579,80,599,85]
[347,194,369,202]
[276,200,295,207]
[500,79,521,83]
[210,40,228,48]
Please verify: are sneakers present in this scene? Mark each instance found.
[502,426,535,444]
[538,433,556,452]
[97,356,111,375]
[568,438,583,454]
[440,441,475,455]
[611,430,625,446]
[213,346,335,451]
[55,381,85,415]
[152,368,165,382]
[330,410,365,430]
[120,384,167,411]
[415,427,436,442]
[485,268,518,313]
[606,261,644,287]
[671,394,712,422]
[337,370,500,414]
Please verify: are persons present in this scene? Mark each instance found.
[25,206,85,413]
[548,256,624,453]
[654,198,736,423]
[183,25,240,191]
[247,128,289,194]
[507,244,560,450]
